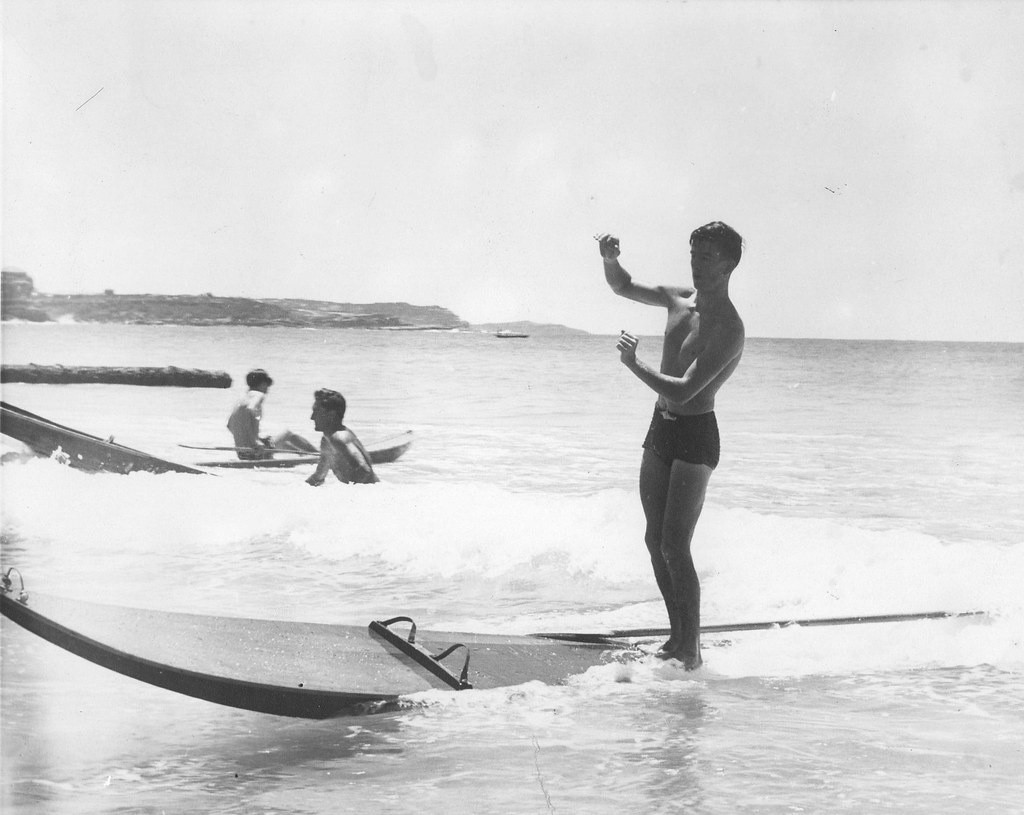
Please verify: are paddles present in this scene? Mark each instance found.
[522,607,988,642]
[178,444,321,456]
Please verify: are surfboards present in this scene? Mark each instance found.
[0,401,218,475]
[194,429,413,468]
[0,566,732,718]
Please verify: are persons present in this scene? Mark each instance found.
[306,388,380,486]
[594,222,744,671]
[227,368,318,460]
